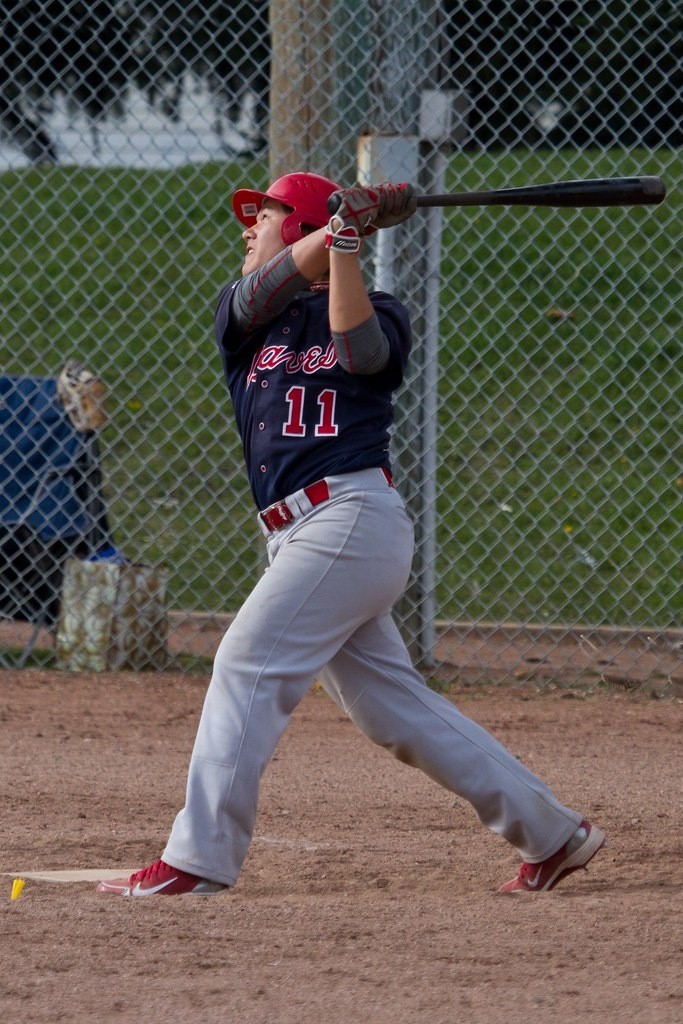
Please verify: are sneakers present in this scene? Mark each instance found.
[93,860,231,899]
[493,818,607,892]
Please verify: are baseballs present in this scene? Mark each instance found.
[325,176,668,220]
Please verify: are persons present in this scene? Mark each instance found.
[96,170,606,901]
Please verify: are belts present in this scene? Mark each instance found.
[260,466,397,533]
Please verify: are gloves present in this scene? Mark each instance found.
[362,183,418,236]
[324,181,382,253]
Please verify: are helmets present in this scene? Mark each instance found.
[232,172,344,247]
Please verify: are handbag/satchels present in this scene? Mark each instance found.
[53,545,171,675]
